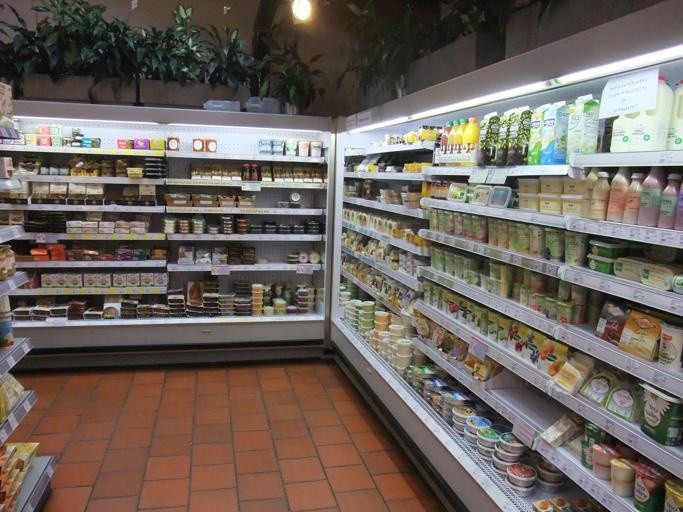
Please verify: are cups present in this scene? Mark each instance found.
[479,260,602,326]
[580,421,683,511]
[657,319,683,370]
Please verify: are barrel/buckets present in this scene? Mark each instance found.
[433,116,480,167]
[609,75,683,153]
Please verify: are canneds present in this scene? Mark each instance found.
[193,138,204,152]
[0,245,16,280]
[206,138,216,152]
[167,137,179,150]
[0,294,14,349]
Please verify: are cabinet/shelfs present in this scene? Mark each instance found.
[0,132,57,510]
[408,147,681,509]
[337,140,437,381]
[7,140,166,318]
[166,151,332,323]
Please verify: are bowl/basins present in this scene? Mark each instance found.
[451,405,566,497]
[338,281,425,378]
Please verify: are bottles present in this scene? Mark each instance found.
[243,163,258,181]
[581,166,683,231]
[431,179,449,200]
[417,126,441,142]
[0,296,14,347]
[438,116,481,167]
